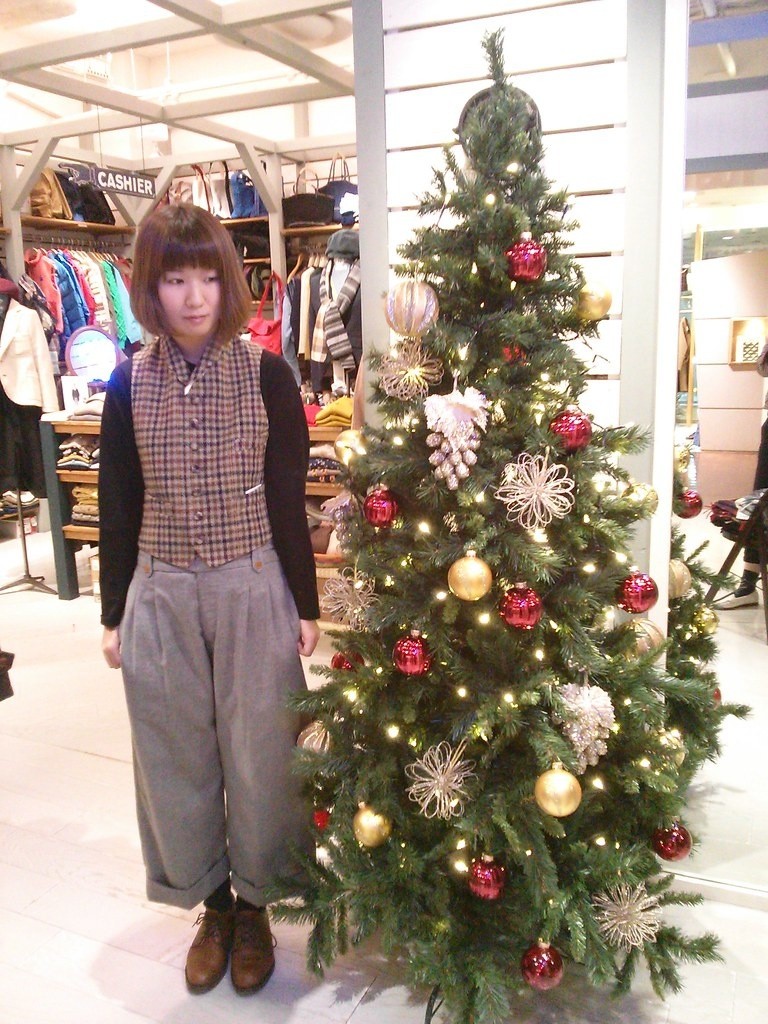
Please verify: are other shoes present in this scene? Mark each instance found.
[709,587,759,608]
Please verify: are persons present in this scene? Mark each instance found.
[91,201,322,997]
[306,228,362,399]
[715,383,768,610]
[1,277,60,510]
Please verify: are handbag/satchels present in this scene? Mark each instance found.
[282,166,335,227]
[28,166,72,219]
[248,273,284,360]
[158,158,268,219]
[317,151,358,225]
[53,169,117,223]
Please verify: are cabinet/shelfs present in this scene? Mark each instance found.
[38,420,350,600]
[220,214,274,306]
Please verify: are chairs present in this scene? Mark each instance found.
[703,490,768,645]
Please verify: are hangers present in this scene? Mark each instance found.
[313,242,321,268]
[287,245,307,284]
[308,244,315,269]
[32,235,118,263]
[318,242,329,268]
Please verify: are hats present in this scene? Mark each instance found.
[324,229,360,259]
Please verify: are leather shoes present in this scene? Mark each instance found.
[232,896,276,995]
[185,892,236,994]
